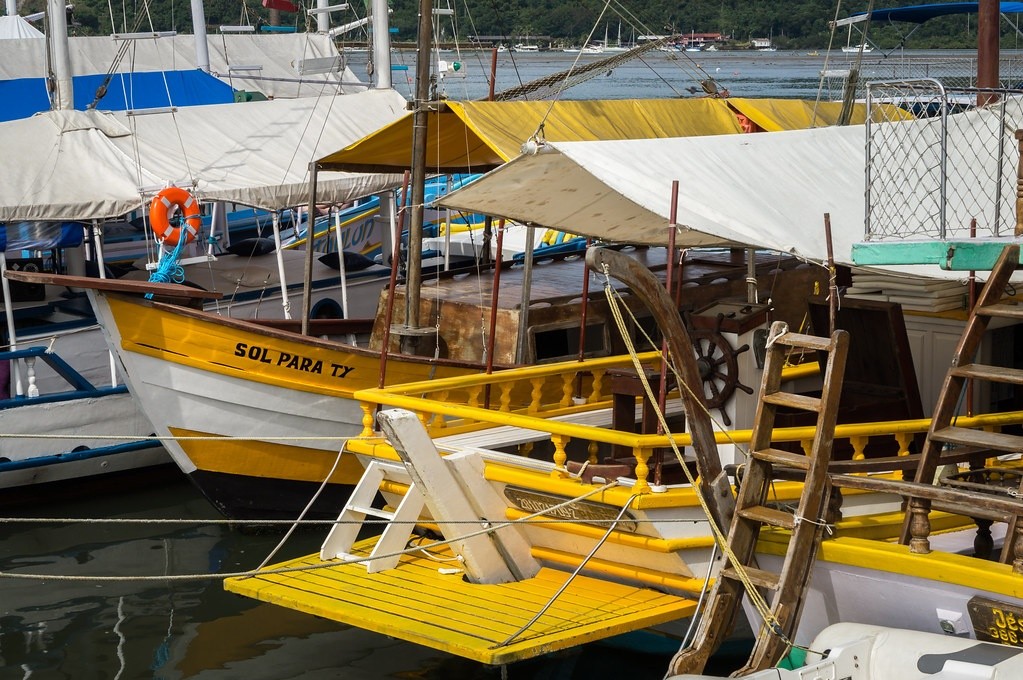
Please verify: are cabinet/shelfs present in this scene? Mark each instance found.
[900,307,1023,431]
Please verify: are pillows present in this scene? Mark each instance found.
[318,252,378,272]
[225,238,276,257]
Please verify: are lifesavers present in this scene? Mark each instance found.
[150,188,201,246]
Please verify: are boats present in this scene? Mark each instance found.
[0,0,1023,680]
